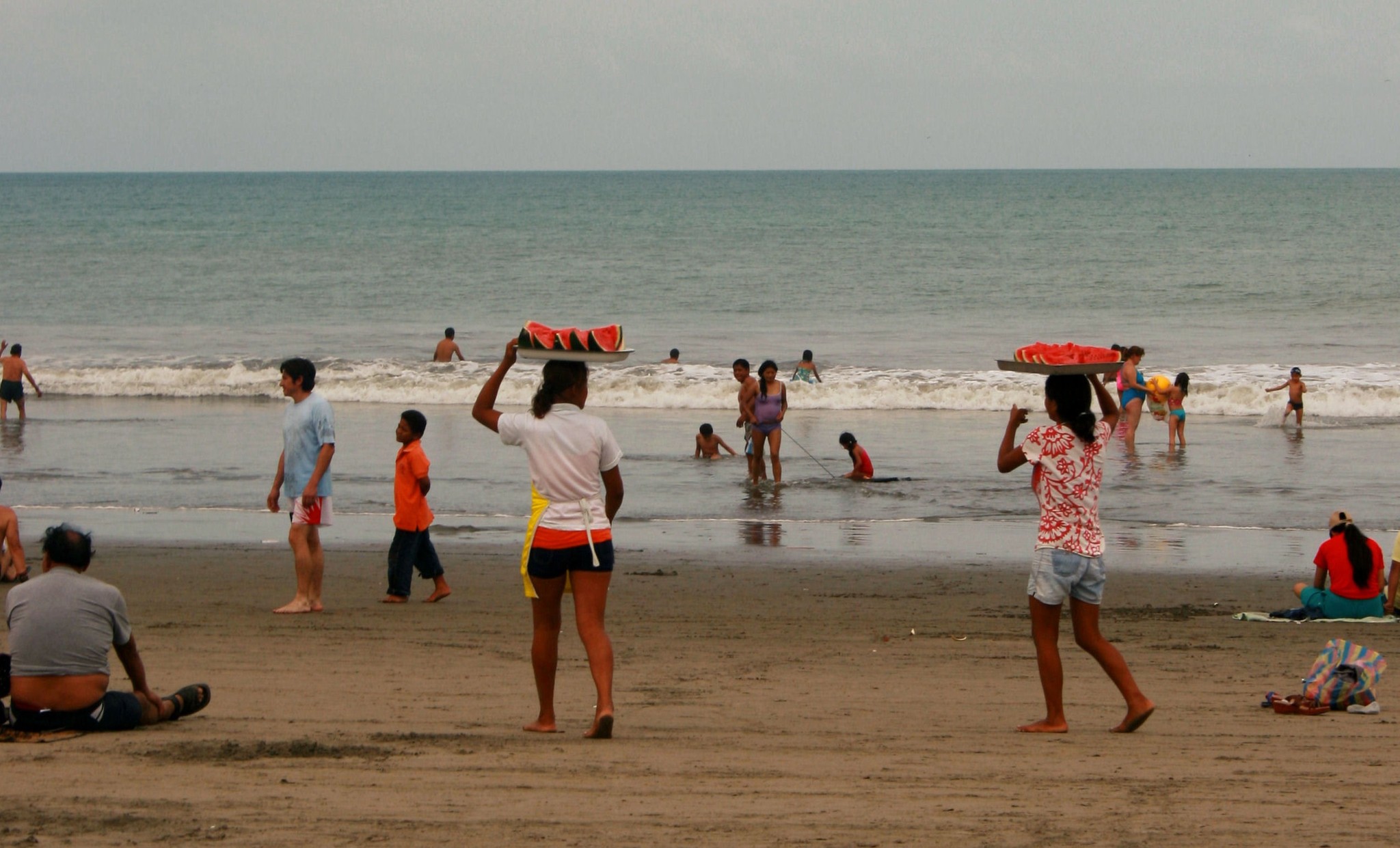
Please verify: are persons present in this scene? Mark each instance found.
[1150,372,1190,456]
[838,432,873,481]
[732,359,758,482]
[378,410,452,604]
[1120,345,1157,456]
[266,358,336,615]
[997,372,1155,734]
[790,349,821,385]
[471,339,624,740]
[740,360,788,486]
[6,521,211,733]
[0,478,31,584]
[433,327,464,362]
[691,423,736,459]
[661,348,680,364]
[1293,510,1386,619]
[1102,343,1129,418]
[1265,367,1307,428]
[0,339,42,422]
[1383,531,1400,620]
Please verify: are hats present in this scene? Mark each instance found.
[1328,510,1353,537]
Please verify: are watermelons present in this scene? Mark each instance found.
[1014,341,1122,365]
[517,320,623,352]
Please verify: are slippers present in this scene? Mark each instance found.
[1264,690,1330,716]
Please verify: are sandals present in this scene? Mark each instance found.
[161,683,211,722]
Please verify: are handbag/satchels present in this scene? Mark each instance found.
[1301,637,1388,710]
[1268,603,1326,621]
